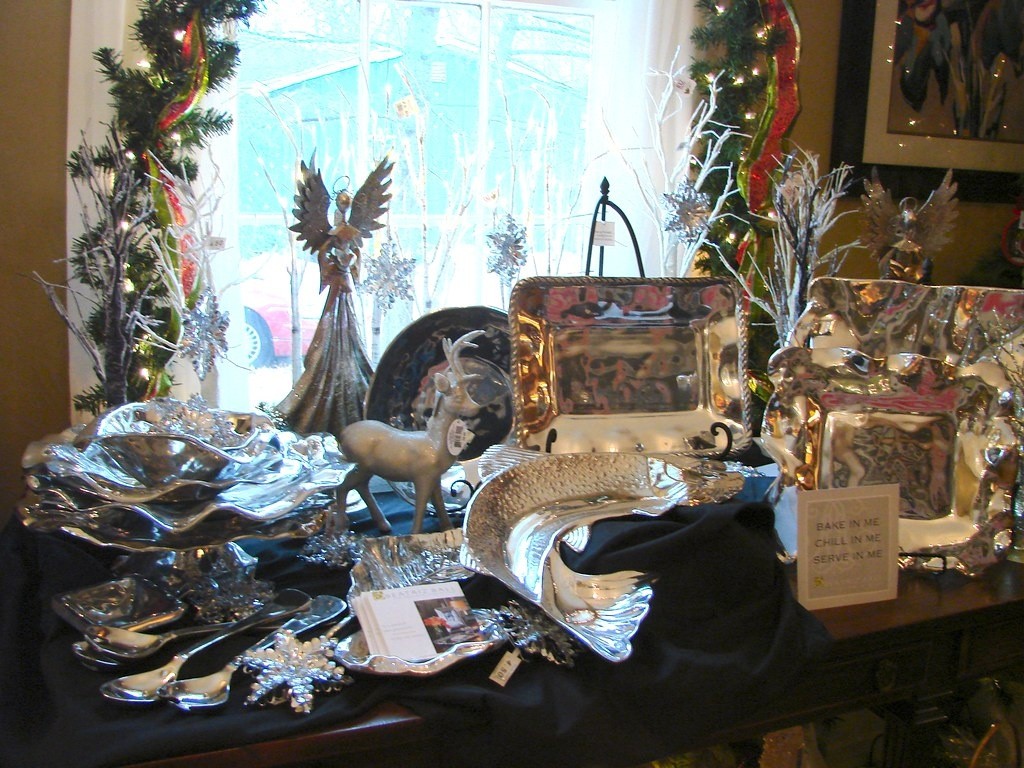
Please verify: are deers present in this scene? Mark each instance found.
[334,329,483,535]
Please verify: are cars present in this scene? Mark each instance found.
[241,259,325,369]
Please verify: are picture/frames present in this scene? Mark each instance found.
[831,0,1024,201]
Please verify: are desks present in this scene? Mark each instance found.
[0,492,1024,768]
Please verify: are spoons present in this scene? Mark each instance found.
[84,621,283,658]
[156,596,346,710]
[99,591,312,704]
[71,620,338,667]
